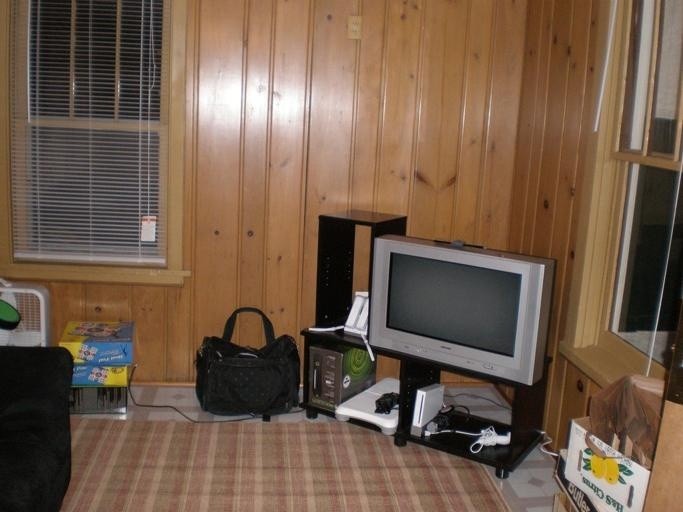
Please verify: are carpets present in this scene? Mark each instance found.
[58,411,510,511]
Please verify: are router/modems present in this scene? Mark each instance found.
[413,383,445,427]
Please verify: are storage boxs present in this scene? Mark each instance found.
[552,413,651,511]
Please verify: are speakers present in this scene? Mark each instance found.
[309,342,377,411]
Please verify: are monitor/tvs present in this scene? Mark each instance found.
[368,234,558,387]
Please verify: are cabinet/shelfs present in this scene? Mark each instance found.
[296,325,553,477]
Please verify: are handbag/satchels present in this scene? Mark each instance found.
[194,306,301,417]
